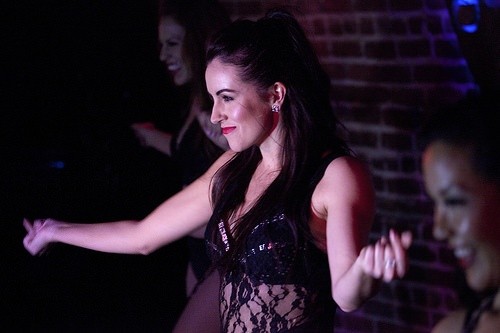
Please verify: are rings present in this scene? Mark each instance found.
[385,259,396,268]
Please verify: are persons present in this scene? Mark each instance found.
[23,11,413,333]
[422,89,500,333]
[129,0,233,333]
